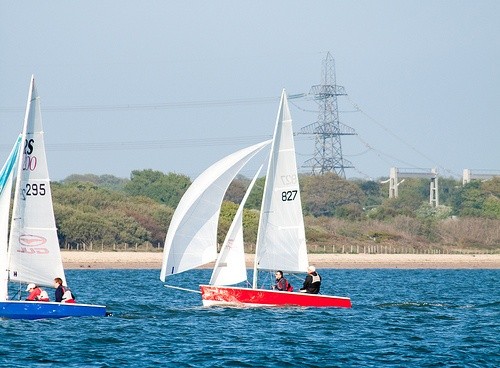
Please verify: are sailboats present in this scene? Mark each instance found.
[0,73,112,322]
[159,87,353,309]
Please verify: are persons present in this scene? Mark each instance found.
[300,266,322,294]
[55,278,75,302]
[273,270,294,292]
[25,283,49,301]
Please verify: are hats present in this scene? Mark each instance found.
[308,265,315,271]
[25,283,36,291]
